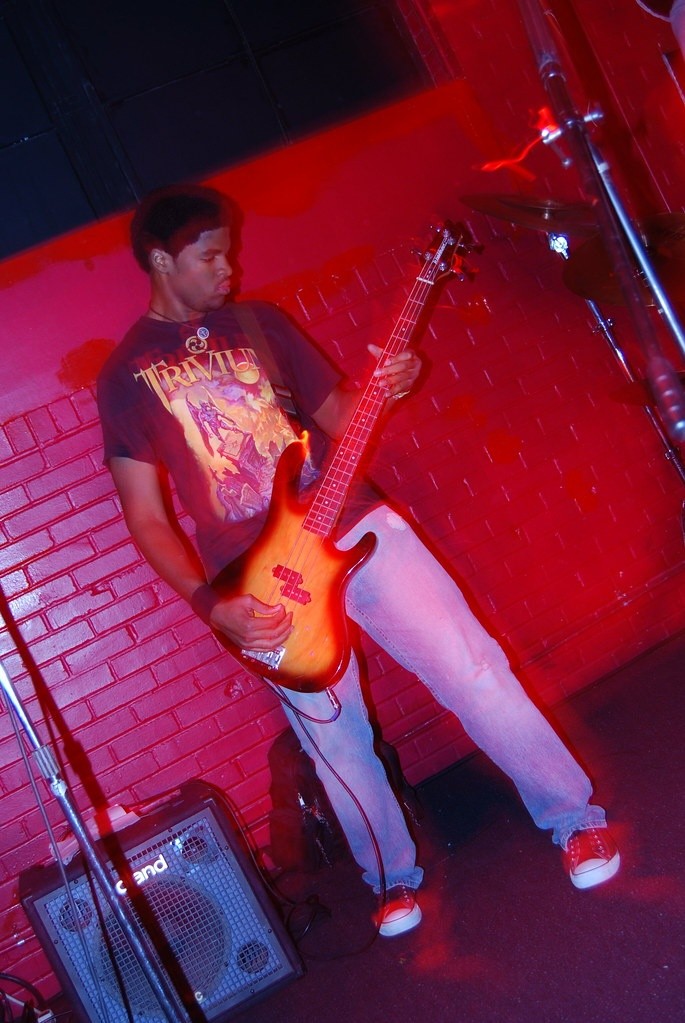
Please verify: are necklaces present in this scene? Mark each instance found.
[144,307,213,340]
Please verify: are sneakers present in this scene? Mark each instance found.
[375,884,421,936]
[567,828,620,888]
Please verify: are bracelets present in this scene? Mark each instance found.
[192,584,220,625]
[386,372,410,400]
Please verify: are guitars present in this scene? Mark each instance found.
[207,218,477,694]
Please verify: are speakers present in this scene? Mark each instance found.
[18,777,307,1023]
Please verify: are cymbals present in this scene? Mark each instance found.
[562,212,685,310]
[454,193,606,234]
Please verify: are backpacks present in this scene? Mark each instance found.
[291,729,424,865]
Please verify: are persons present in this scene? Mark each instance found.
[94,184,622,936]
